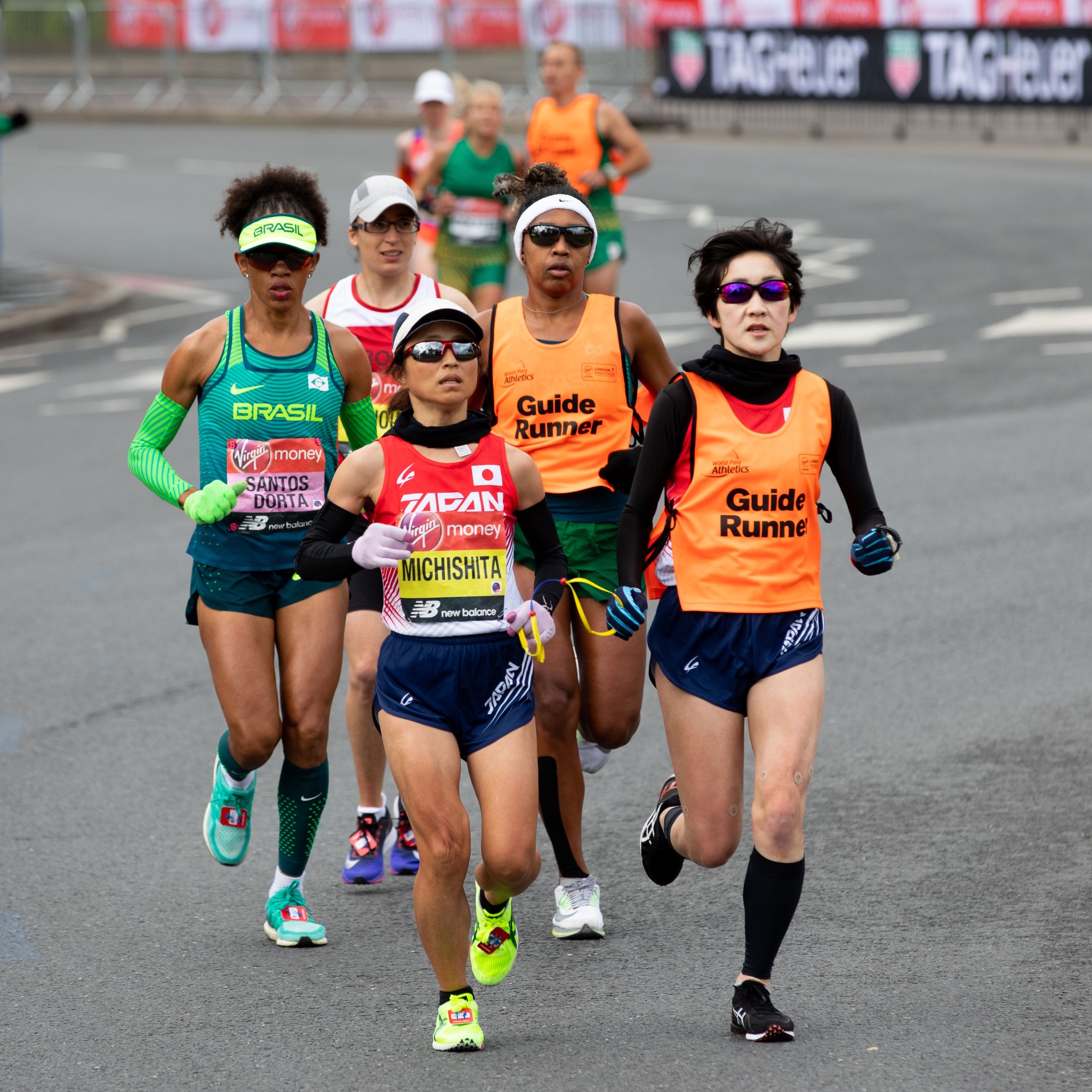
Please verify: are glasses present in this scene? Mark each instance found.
[523,224,595,249]
[245,246,314,270]
[352,218,420,232]
[405,340,482,363]
[718,280,794,304]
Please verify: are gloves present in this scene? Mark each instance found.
[350,522,413,569]
[850,524,902,576]
[507,596,557,649]
[599,446,642,496]
[605,586,648,639]
[184,477,247,522]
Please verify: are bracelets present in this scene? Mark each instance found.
[603,163,621,181]
[420,194,435,216]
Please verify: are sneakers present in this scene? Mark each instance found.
[727,978,795,1041]
[552,876,606,938]
[342,807,392,885]
[432,992,485,1051]
[574,729,610,774]
[262,881,327,947]
[640,774,685,884]
[203,754,257,867]
[389,795,420,875]
[469,880,520,986]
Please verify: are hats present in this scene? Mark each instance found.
[391,297,484,355]
[234,215,317,255]
[349,175,420,222]
[413,70,454,108]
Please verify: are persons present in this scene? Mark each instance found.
[393,68,469,283]
[441,163,689,941]
[591,216,905,1041]
[411,78,519,312]
[303,175,483,886]
[126,157,378,941]
[502,38,649,301]
[293,306,569,1053]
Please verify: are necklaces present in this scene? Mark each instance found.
[523,292,585,313]
